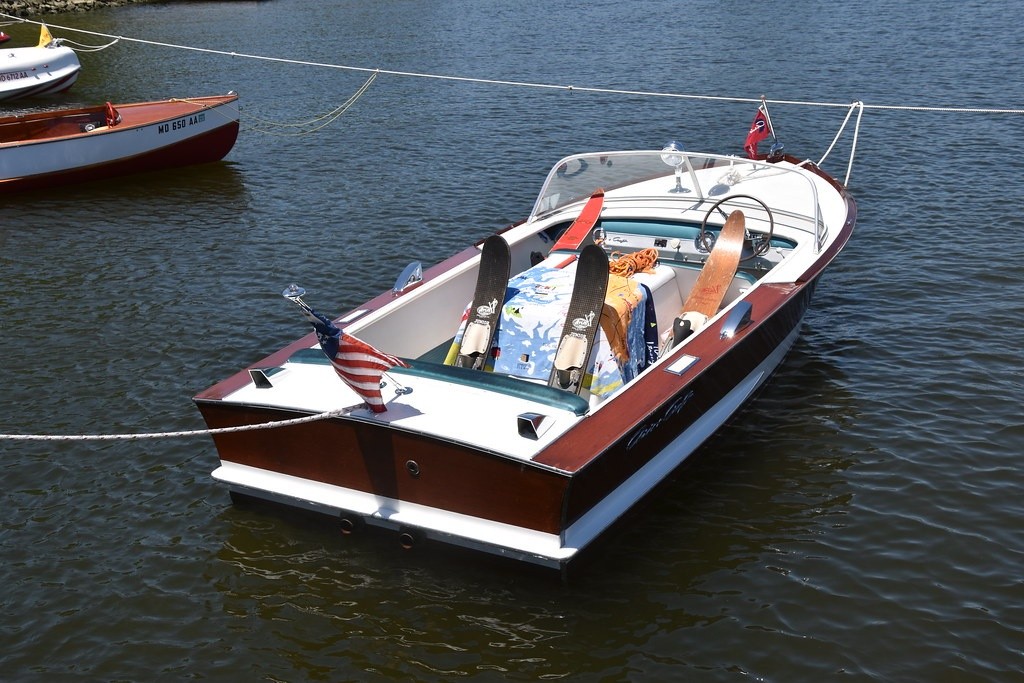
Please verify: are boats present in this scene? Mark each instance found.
[0,21,82,103]
[0,90,240,197]
[190,138,858,572]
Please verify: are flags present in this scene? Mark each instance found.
[38,26,52,47]
[744,105,771,158]
[300,304,413,413]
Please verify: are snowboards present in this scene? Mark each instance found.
[657,209,748,361]
[547,188,606,251]
[451,233,512,372]
[546,243,610,399]
